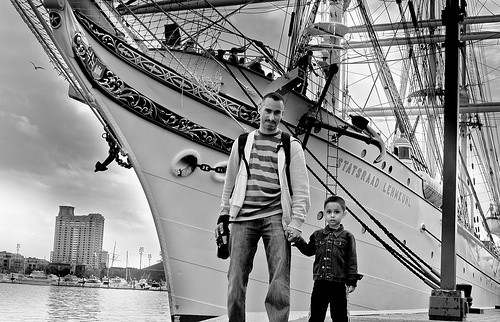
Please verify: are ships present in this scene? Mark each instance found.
[9,0,500,322]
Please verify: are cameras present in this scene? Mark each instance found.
[216,230,230,259]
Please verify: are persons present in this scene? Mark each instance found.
[214,92,311,322]
[288,196,364,322]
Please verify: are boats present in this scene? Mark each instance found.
[1,271,167,291]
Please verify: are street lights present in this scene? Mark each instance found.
[137,245,145,275]
[147,253,152,269]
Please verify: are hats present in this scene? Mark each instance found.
[252,56,264,62]
